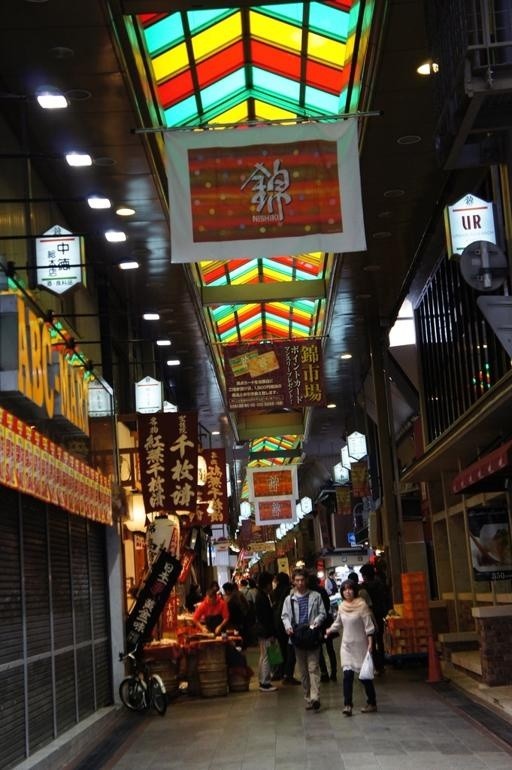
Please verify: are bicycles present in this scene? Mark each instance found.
[115,640,167,715]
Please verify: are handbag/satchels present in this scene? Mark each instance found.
[290,619,323,651]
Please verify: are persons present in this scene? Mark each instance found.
[307,575,337,683]
[256,572,279,692]
[186,573,280,656]
[360,563,389,675]
[348,572,373,609]
[325,580,377,716]
[324,570,337,596]
[270,573,302,685]
[281,569,328,710]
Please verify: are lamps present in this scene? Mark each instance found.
[1,82,145,275]
[45,307,183,369]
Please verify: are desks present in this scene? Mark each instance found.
[144,633,242,696]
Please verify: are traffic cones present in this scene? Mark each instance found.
[424,635,451,684]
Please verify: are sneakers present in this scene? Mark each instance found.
[374,670,387,682]
[320,671,338,682]
[306,700,320,712]
[258,682,277,692]
[361,704,377,713]
[266,670,301,686]
[343,705,353,715]
